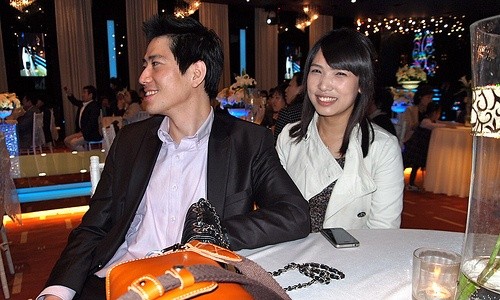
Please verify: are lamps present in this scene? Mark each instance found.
[266,17,277,25]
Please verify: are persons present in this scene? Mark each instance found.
[365,84,456,186]
[276,28,404,233]
[16,77,145,151]
[36,14,311,300]
[260,72,304,140]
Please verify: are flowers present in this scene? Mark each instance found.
[397,64,427,82]
[219,68,257,109]
[390,87,415,106]
[0,93,22,110]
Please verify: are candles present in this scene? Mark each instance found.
[425,282,452,300]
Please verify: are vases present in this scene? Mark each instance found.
[457,16,500,297]
[0,110,12,124]
[401,80,421,90]
[244,88,260,122]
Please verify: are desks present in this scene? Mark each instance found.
[0,136,21,300]
[422,125,500,200]
[231,229,500,300]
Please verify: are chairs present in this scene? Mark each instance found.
[18,108,115,194]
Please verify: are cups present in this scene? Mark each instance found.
[412,247,462,300]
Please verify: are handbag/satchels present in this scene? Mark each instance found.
[180,198,233,251]
[106,239,291,300]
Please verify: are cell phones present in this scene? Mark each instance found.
[321,227,360,248]
